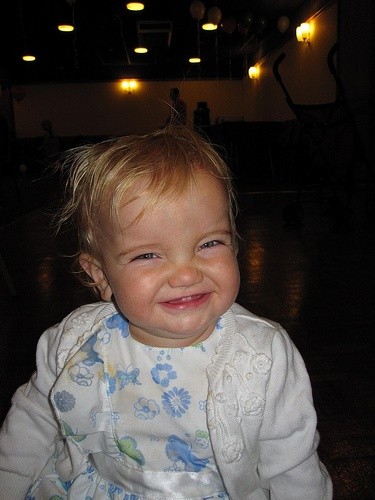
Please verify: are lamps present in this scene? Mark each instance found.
[296,23,312,48]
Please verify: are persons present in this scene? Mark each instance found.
[33,120,54,174]
[169,88,187,129]
[0,130,333,500]
